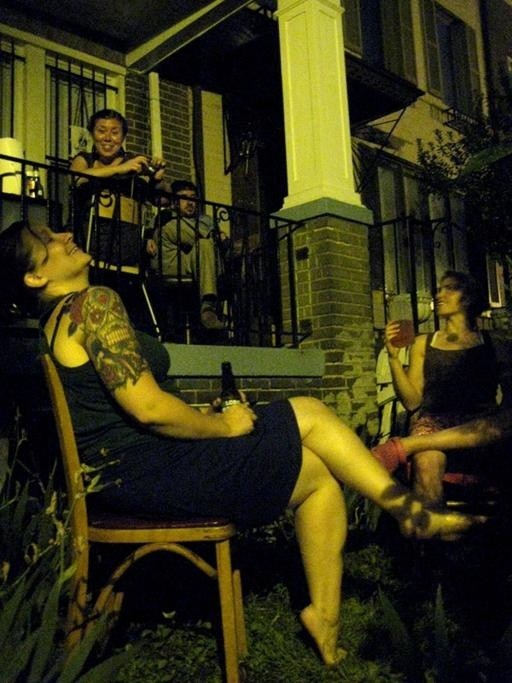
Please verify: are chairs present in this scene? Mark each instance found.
[0,327,255,682]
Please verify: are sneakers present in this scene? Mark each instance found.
[200,303,222,329]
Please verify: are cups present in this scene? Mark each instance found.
[386,292,416,348]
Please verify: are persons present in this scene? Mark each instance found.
[0,216,491,667]
[369,268,511,544]
[141,179,229,334]
[66,110,168,331]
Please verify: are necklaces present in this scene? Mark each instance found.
[446,332,460,342]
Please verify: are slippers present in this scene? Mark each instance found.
[370,436,409,475]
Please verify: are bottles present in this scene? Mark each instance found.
[217,360,247,411]
[28,164,44,201]
